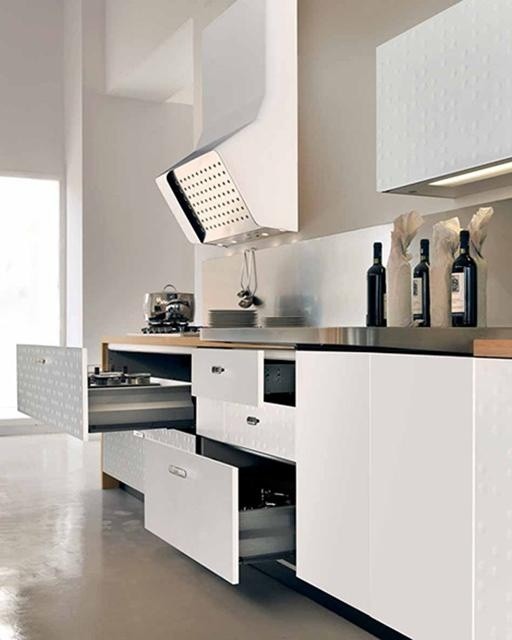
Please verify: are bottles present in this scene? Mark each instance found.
[410,237,432,325]
[451,230,480,328]
[365,241,391,327]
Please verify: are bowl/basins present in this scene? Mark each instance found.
[93,371,152,386]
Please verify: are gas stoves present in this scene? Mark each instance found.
[128,324,202,339]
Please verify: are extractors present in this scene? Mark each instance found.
[155,1,300,249]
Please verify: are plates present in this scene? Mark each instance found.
[207,308,305,327]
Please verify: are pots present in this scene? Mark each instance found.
[143,284,195,323]
